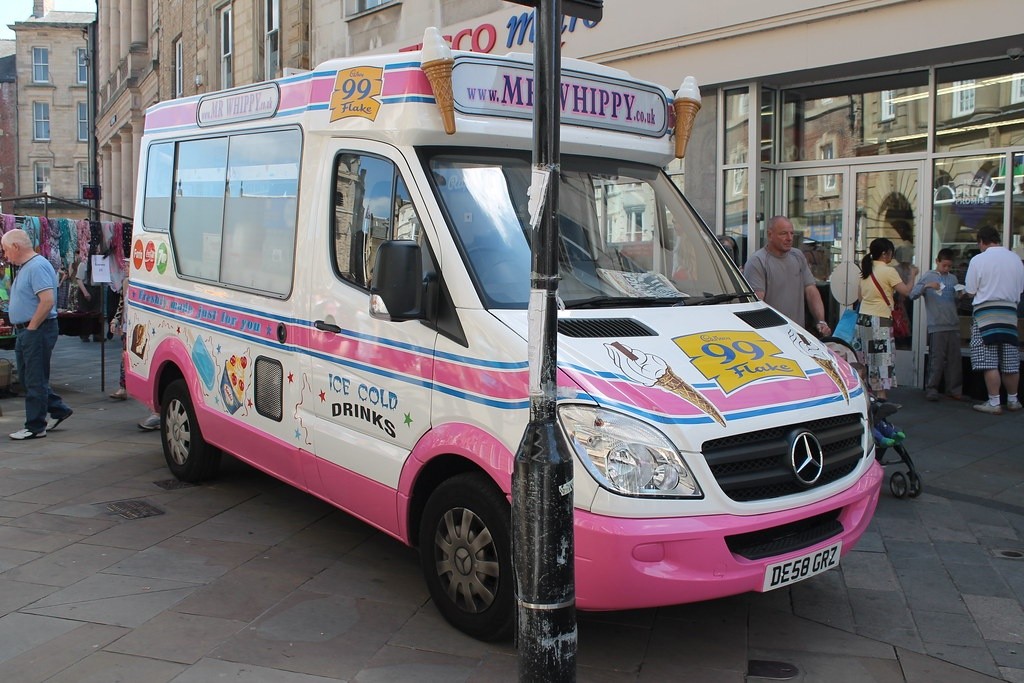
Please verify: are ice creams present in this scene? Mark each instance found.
[674,76,702,158]
[421,26,457,135]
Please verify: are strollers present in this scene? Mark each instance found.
[818,336,921,498]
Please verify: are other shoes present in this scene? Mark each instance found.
[927,393,938,401]
[951,393,963,400]
[109,388,127,400]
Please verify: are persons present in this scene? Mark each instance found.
[856,237,920,408]
[57,268,65,291]
[962,225,1024,415]
[137,412,161,430]
[108,258,129,401]
[717,234,742,273]
[1,229,73,439]
[105,253,128,339]
[744,215,832,336]
[671,216,698,283]
[909,248,969,401]
[850,361,906,447]
[0,261,11,325]
[77,255,106,340]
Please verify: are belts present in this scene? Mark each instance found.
[16,320,30,329]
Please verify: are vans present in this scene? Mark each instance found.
[123,25,883,642]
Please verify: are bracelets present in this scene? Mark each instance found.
[816,320,827,325]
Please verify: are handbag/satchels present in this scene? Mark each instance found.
[831,303,861,346]
[890,300,911,339]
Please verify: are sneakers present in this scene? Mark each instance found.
[1006,399,1022,410]
[8,428,47,440]
[138,414,161,429]
[45,406,73,430]
[972,399,1003,415]
